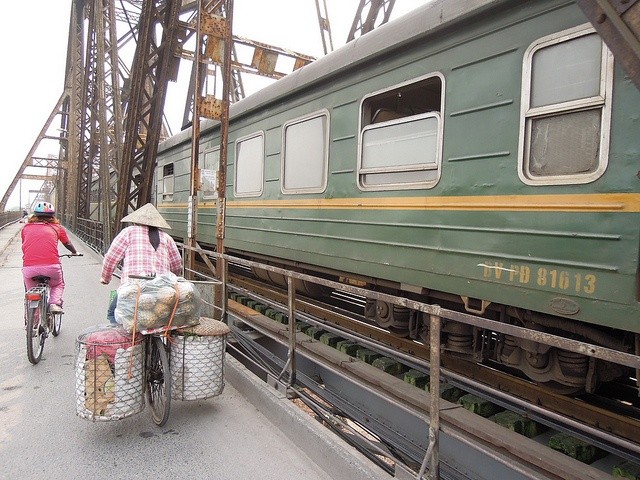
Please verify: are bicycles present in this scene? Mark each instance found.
[21,254,84,363]
[101,273,171,426]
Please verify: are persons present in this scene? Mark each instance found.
[21,203,78,336]
[101,203,182,292]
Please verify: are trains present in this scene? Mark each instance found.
[90,1,639,478]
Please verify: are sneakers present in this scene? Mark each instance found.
[50,303,64,312]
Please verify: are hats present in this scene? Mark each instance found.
[121,202,173,231]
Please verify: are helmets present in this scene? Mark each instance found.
[34,202,56,214]
